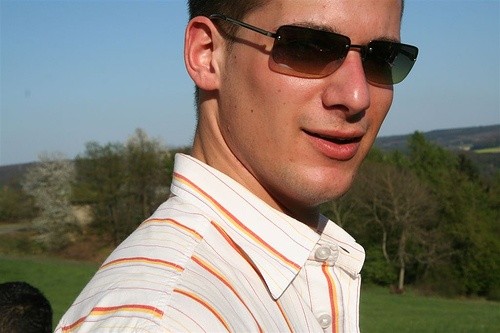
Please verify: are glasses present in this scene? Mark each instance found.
[210,13,421,86]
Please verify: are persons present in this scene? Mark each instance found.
[0,281,52,333]
[52,0,418,332]
